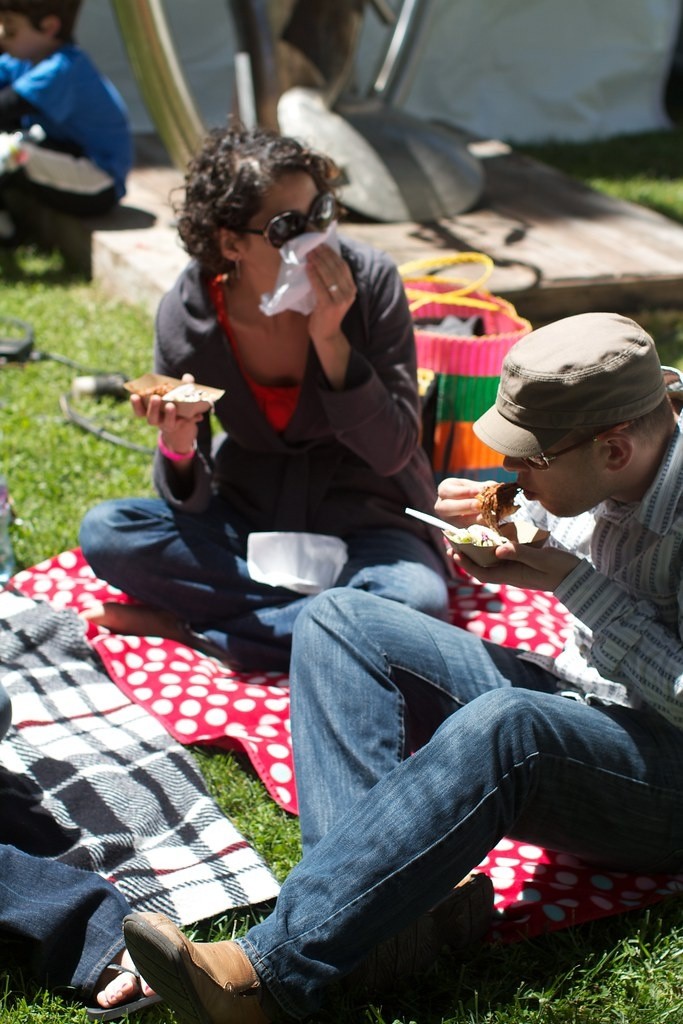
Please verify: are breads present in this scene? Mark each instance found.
[478,480,521,530]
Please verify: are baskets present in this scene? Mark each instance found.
[398,254,532,487]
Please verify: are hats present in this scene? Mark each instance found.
[472,313,665,458]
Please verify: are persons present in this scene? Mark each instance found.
[0,0,134,279]
[0,678,161,1022]
[123,309,683,1024]
[77,112,455,668]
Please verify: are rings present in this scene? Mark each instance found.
[328,286,338,292]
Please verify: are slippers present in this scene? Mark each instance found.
[86,960,165,1023]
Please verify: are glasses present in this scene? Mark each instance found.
[236,193,336,248]
[521,419,634,472]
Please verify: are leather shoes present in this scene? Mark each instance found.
[348,872,496,993]
[122,910,271,1024]
